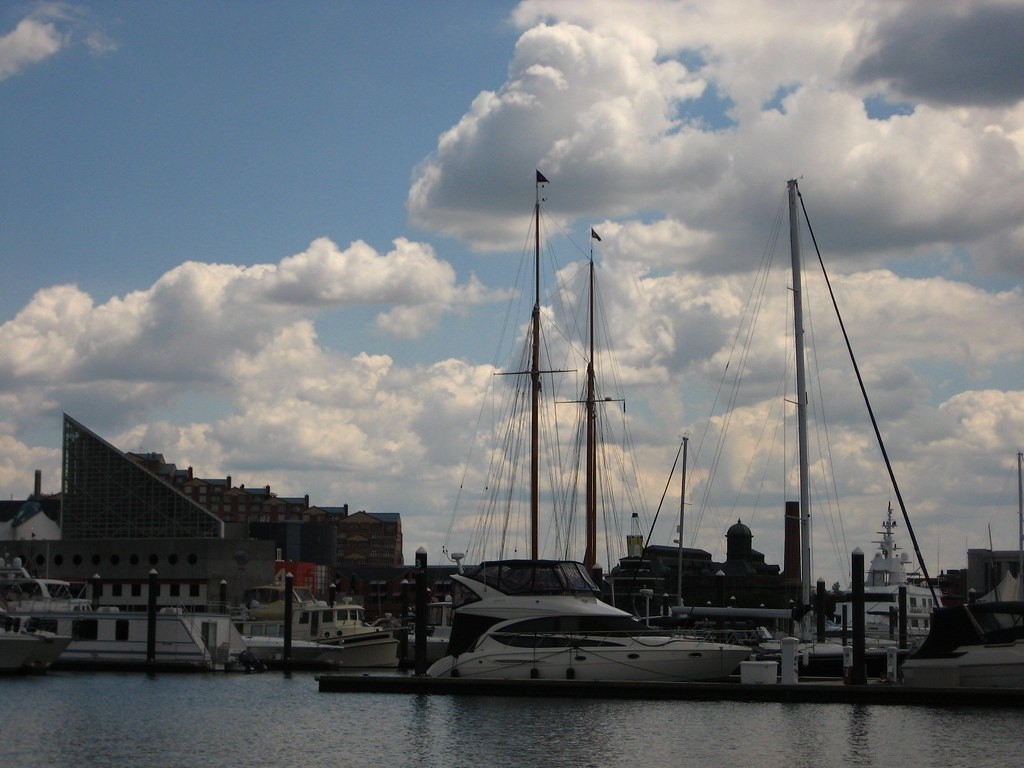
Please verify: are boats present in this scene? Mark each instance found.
[0,163,1024,702]
